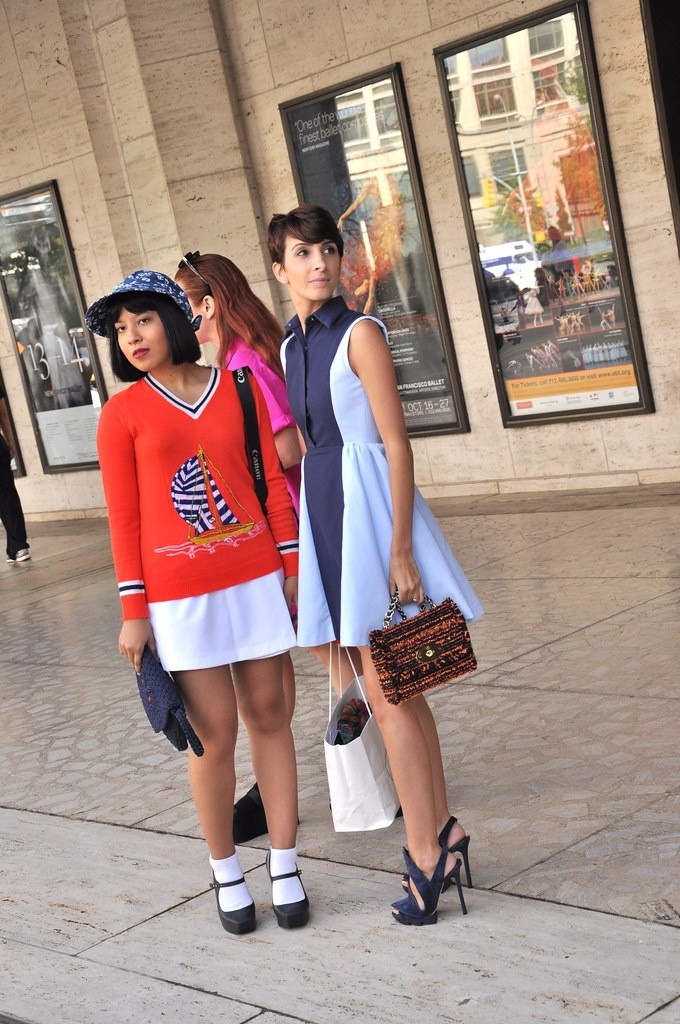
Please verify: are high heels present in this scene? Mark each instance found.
[209,870,256,934]
[265,852,309,928]
[391,845,468,926]
[402,816,472,892]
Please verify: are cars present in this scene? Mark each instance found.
[486,274,532,331]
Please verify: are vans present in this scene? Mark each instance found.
[479,240,540,306]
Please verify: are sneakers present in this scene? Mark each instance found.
[6,548,31,562]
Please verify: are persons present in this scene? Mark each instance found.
[606,264,619,288]
[525,340,561,371]
[546,226,591,319]
[533,267,556,305]
[582,339,627,365]
[597,304,616,330]
[0,367,31,563]
[267,205,485,926]
[175,251,364,720]
[85,268,309,935]
[547,259,609,301]
[13,248,88,405]
[554,310,586,335]
[523,281,547,327]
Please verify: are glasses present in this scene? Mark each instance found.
[178,250,208,285]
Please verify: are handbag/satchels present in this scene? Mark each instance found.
[366,590,477,705]
[133,648,204,760]
[323,639,399,833]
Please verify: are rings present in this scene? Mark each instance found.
[413,597,418,601]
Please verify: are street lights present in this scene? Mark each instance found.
[493,93,533,246]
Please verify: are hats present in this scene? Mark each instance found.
[85,270,202,337]
